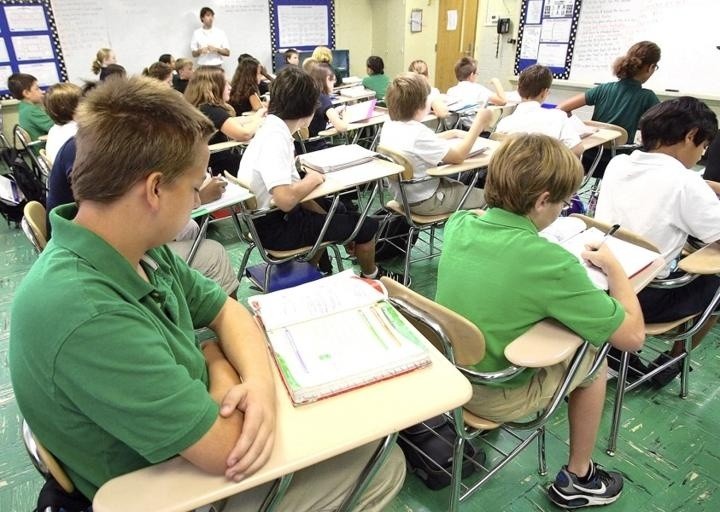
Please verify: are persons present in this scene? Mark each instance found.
[9,73,407,511]
[191,8,230,70]
[432,131,624,509]
[7,42,660,298]
[594,95,720,389]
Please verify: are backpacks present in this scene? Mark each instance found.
[396,413,487,491]
[0,147,46,224]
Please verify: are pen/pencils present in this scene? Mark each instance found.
[303,168,308,174]
[582,224,620,263]
[208,166,213,178]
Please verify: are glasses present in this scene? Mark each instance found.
[561,198,574,212]
[652,64,659,70]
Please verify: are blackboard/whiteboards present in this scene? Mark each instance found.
[49,0,273,89]
[509,0,720,101]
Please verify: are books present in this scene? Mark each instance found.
[251,277,431,408]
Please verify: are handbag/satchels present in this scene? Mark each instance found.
[370,206,420,267]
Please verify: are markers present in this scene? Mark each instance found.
[665,89,679,92]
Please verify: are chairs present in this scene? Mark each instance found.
[293,127,312,157]
[223,169,343,294]
[582,119,641,155]
[33,148,54,187]
[18,420,93,511]
[19,201,50,259]
[373,146,459,287]
[568,212,688,457]
[380,273,548,512]
[9,123,46,177]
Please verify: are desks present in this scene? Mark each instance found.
[330,87,377,106]
[571,126,622,191]
[657,239,720,355]
[503,256,662,431]
[179,184,252,268]
[487,99,518,133]
[317,107,388,149]
[291,154,406,261]
[420,108,458,130]
[426,128,498,228]
[92,301,475,512]
[201,138,248,151]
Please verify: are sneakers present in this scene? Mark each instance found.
[647,350,693,390]
[360,264,412,288]
[606,347,648,386]
[315,253,333,278]
[547,459,624,511]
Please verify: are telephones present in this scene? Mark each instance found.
[497,18,510,34]
